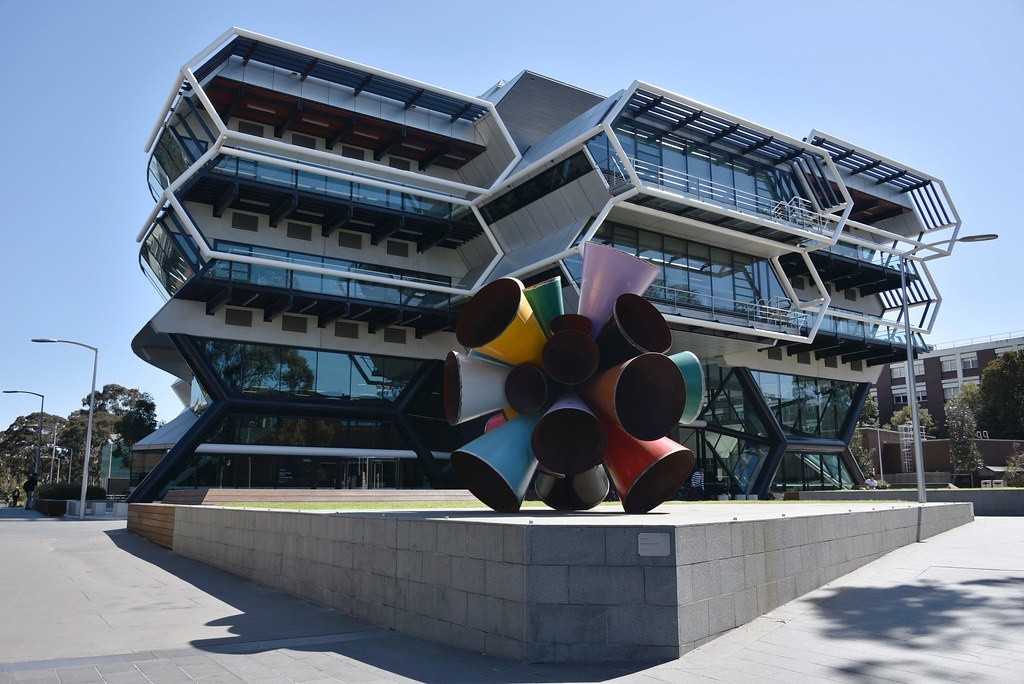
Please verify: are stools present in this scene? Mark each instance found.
[68,500,127,515]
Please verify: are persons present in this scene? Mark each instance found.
[23,472,36,510]
[864,475,878,490]
[12,487,19,507]
[691,468,704,502]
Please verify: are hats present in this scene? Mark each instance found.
[33,473,38,475]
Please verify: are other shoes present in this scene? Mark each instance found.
[25,507,32,510]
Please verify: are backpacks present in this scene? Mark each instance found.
[23,481,31,490]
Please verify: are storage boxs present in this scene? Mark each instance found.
[981,479,1003,488]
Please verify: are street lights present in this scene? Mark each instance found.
[3,388,45,476]
[30,336,98,521]
[897,233,999,513]
